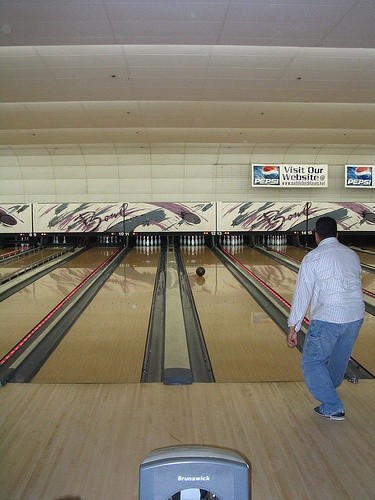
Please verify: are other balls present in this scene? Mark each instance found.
[196,267,205,276]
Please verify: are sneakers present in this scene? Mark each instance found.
[313,406,346,420]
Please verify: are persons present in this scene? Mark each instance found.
[287,217,365,420]
